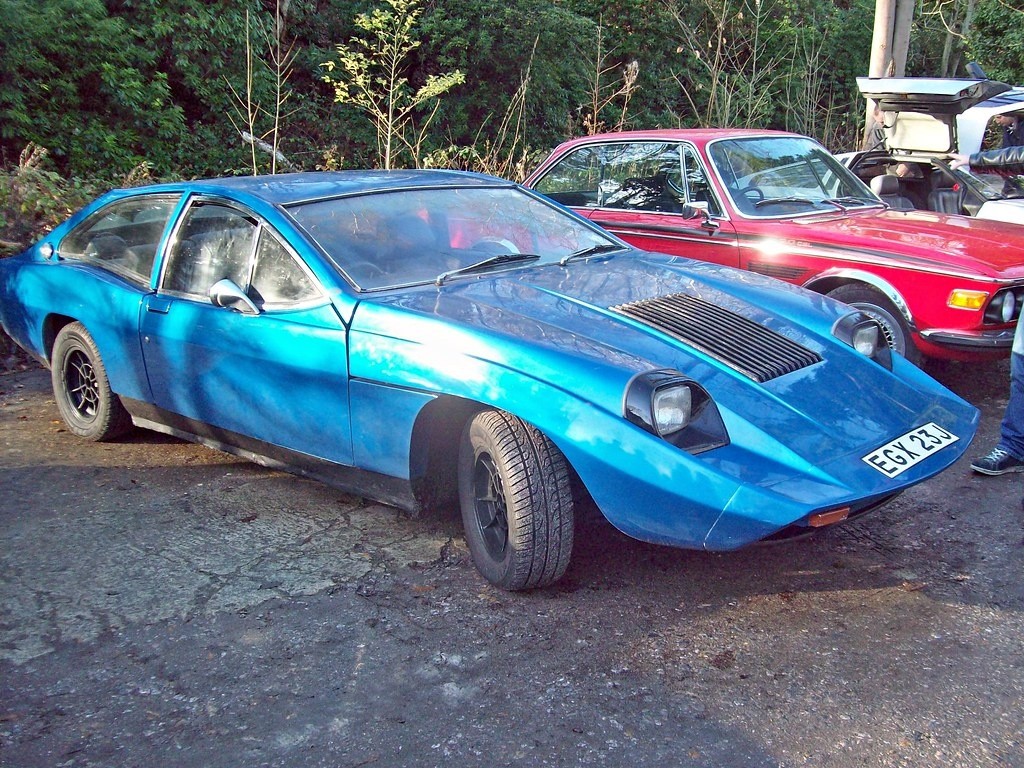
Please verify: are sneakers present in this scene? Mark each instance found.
[970,447,1024,476]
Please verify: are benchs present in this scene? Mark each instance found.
[86,229,252,291]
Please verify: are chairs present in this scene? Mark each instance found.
[869,175,914,209]
[379,213,462,281]
[927,170,969,215]
[697,169,756,217]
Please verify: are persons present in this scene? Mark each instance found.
[970,303,1024,475]
[872,105,923,177]
[995,115,1024,175]
[931,145,1024,176]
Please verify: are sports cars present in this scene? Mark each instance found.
[1,168,984,593]
[730,73,1024,226]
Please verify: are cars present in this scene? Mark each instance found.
[412,128,1024,367]
